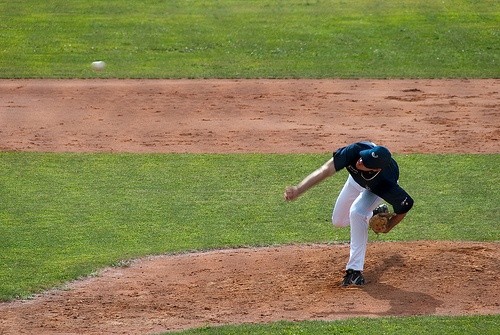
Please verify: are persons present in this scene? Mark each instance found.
[285,140,414,284]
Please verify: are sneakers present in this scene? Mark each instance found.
[342,269,365,287]
[373,204,388,216]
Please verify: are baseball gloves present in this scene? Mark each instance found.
[369,204,391,234]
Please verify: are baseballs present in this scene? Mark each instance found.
[90,60,105,71]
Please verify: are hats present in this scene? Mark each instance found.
[359,146,391,169]
